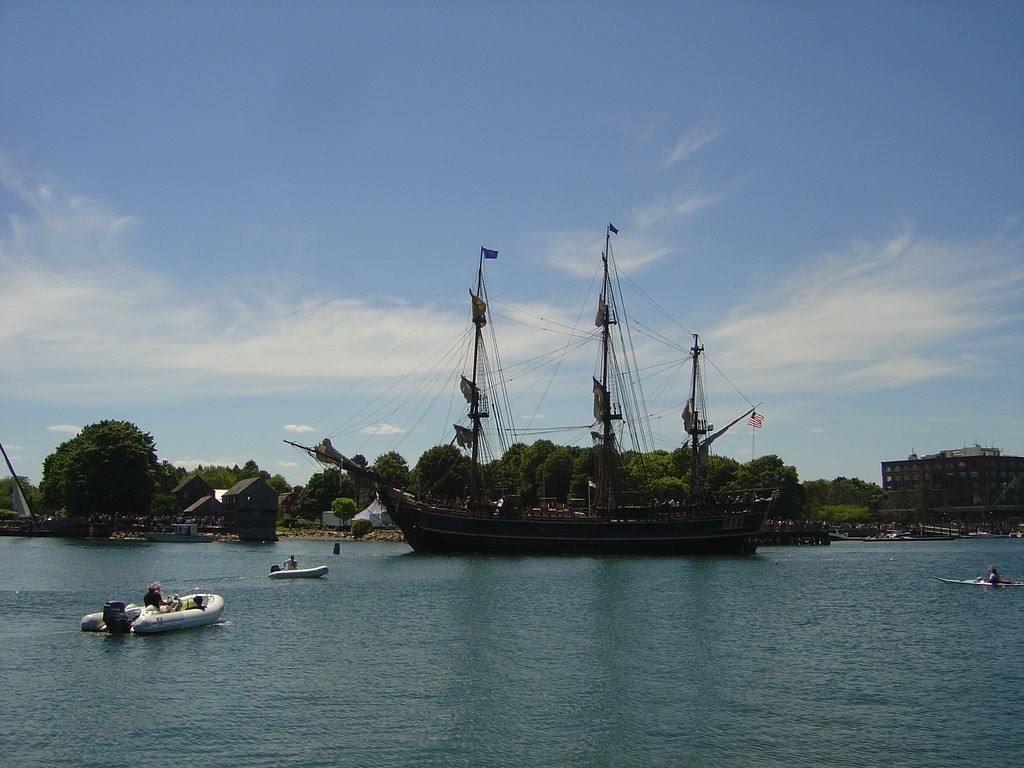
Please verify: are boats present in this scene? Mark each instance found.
[143,524,214,542]
[936,575,1024,587]
[266,564,329,579]
[81,593,225,634]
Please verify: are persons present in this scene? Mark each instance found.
[185,596,205,610]
[977,576,986,584]
[144,581,182,612]
[654,497,686,516]
[767,517,827,530]
[726,493,744,506]
[986,565,999,583]
[282,555,298,570]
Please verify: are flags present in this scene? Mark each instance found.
[483,247,498,259]
[747,411,764,428]
[610,222,619,235]
[589,480,596,488]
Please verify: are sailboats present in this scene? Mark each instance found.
[282,222,790,555]
